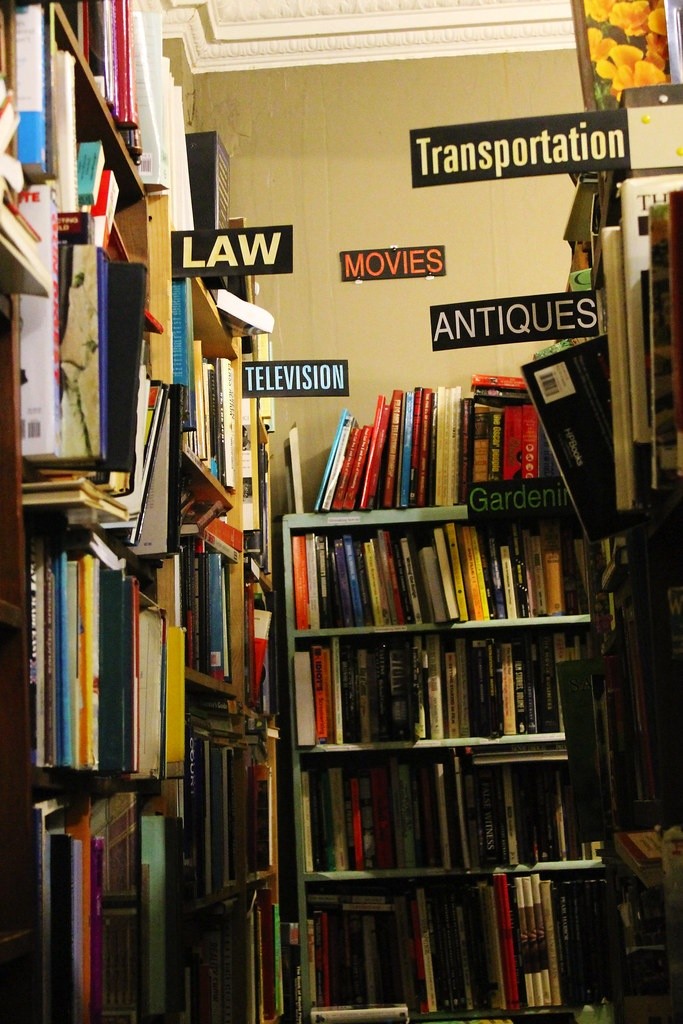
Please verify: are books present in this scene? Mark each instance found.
[284,369,614,1024]
[519,35,683,1022]
[2,0,285,1024]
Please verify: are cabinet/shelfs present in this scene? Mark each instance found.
[573,78,682,1024]
[0,0,276,1024]
[283,495,612,1022]
